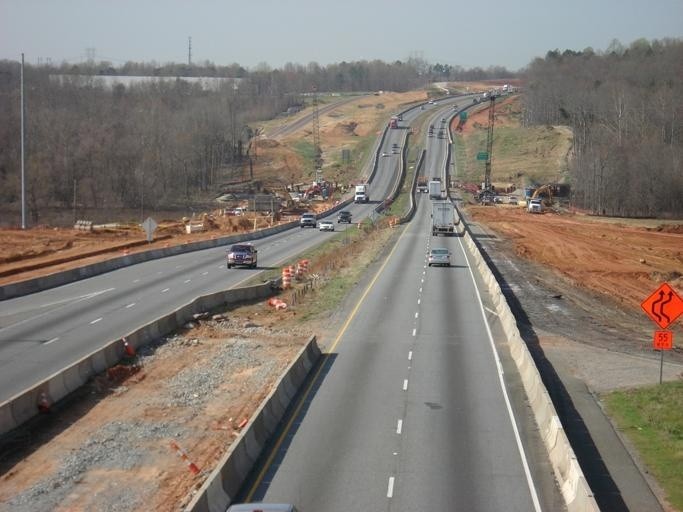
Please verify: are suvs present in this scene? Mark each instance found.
[507,196,517,205]
[337,211,352,224]
[299,213,316,228]
[225,244,257,269]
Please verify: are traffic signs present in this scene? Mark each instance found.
[655,332,672,349]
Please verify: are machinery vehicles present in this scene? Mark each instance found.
[526,185,553,214]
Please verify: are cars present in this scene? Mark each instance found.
[318,221,334,231]
[225,502,298,512]
[234,208,245,215]
[428,248,451,268]
[381,83,520,157]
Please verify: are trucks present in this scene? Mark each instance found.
[428,181,441,200]
[416,176,428,193]
[525,184,571,200]
[353,183,369,204]
[430,202,454,237]
